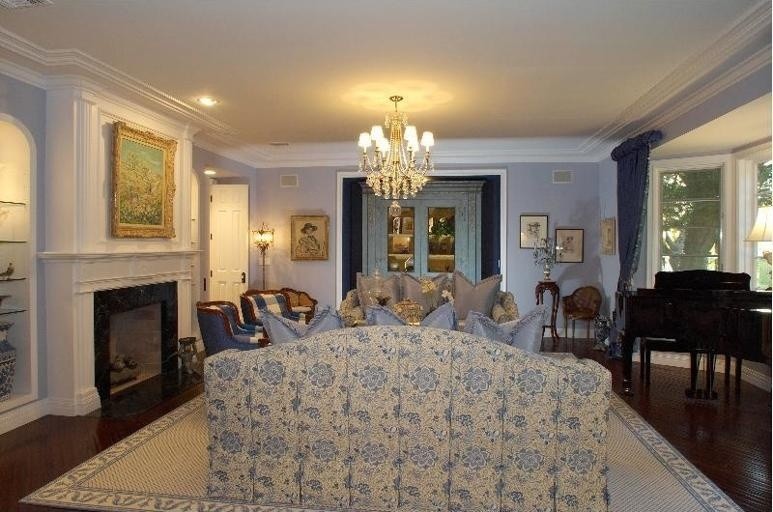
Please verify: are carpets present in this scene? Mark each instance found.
[18,390,746,512]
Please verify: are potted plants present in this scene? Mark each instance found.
[531,238,558,279]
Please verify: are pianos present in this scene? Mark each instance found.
[622,271,771,399]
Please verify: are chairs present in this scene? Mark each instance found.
[562,286,602,344]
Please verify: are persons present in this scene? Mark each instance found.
[298,223,320,255]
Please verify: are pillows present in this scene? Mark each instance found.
[365,304,460,331]
[355,271,400,306]
[464,304,548,354]
[401,273,448,313]
[260,309,342,346]
[452,270,503,321]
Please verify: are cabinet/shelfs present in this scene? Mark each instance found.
[534,280,561,342]
[357,180,487,286]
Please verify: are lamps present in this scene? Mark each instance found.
[252,221,275,290]
[744,205,772,291]
[357,95,434,201]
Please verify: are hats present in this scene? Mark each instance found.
[301,222,317,233]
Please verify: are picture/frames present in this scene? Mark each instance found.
[111,120,179,240]
[600,216,616,256]
[554,228,585,264]
[290,214,330,261]
[519,214,548,250]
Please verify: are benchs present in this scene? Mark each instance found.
[639,337,743,392]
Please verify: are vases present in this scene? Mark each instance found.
[0,320,17,402]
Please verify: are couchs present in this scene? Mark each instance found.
[240,289,308,337]
[339,277,520,332]
[280,287,318,319]
[202,324,612,511]
[195,300,271,357]
